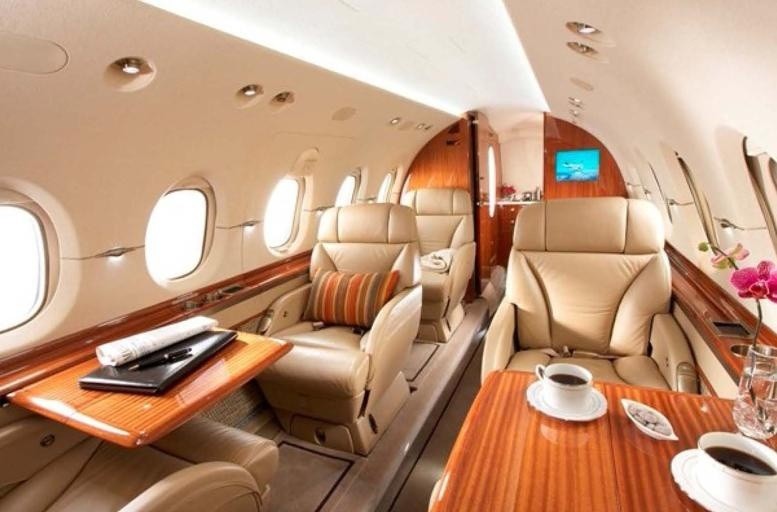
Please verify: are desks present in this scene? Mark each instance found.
[6,322,296,448]
[424,368,775,512]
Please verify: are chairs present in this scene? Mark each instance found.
[0,405,280,512]
[398,187,478,346]
[252,201,426,460]
[474,195,700,399]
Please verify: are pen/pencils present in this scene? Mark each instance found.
[127,347,192,372]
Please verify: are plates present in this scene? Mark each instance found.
[620,397,681,442]
[525,379,609,422]
[671,449,777,511]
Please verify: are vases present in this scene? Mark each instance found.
[728,344,776,442]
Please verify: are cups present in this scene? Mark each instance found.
[534,363,594,409]
[697,431,777,507]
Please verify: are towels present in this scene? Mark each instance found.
[419,244,456,275]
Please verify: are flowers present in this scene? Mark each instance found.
[692,236,776,432]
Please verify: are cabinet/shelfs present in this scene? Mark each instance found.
[497,201,526,271]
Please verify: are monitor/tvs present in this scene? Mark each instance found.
[554,148,601,183]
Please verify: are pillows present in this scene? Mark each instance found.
[300,266,399,333]
